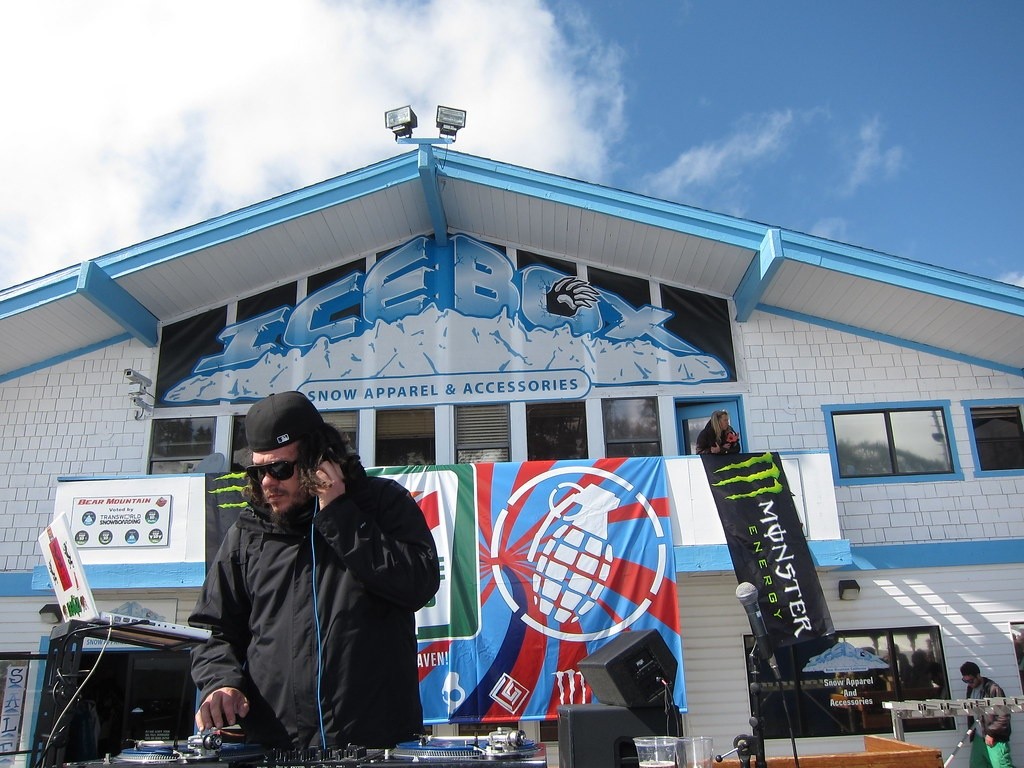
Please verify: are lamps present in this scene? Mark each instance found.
[384,105,418,143]
[839,580,860,601]
[436,105,466,142]
[38,603,63,624]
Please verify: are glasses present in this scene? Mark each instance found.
[962,676,975,684]
[245,460,302,484]
[712,409,728,414]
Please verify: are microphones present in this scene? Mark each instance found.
[735,582,782,681]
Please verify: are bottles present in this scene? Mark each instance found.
[46,527,72,591]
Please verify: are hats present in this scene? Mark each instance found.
[244,391,324,453]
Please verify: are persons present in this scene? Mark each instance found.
[884,644,944,687]
[696,410,741,454]
[187,391,440,753]
[960,662,1011,768]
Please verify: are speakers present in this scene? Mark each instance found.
[576,628,678,708]
[558,702,687,768]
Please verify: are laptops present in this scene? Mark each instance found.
[37,513,212,651]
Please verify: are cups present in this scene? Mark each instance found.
[632,736,679,768]
[674,736,713,768]
